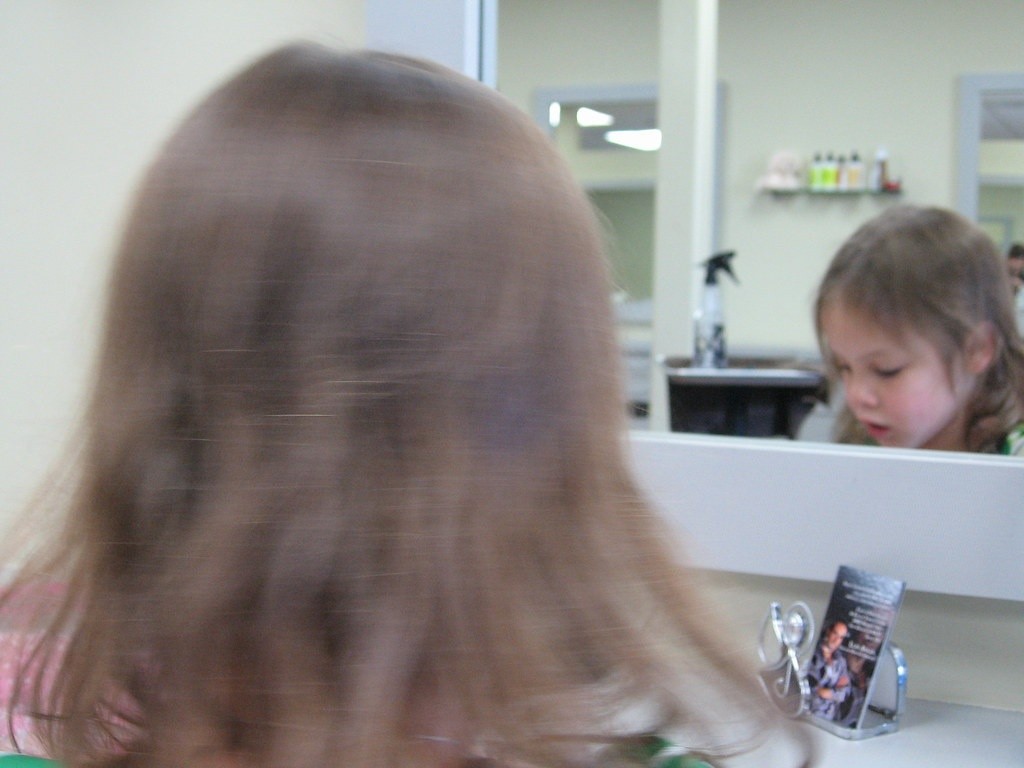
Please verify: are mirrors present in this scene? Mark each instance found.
[462,1,1024,467]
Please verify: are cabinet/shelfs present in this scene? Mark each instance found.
[667,368,829,442]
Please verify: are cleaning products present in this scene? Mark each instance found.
[692,250,742,368]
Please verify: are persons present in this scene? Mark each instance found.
[803,622,850,719]
[1006,245,1024,294]
[0,42,757,768]
[796,204,1024,457]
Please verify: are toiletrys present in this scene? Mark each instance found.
[808,148,900,190]
